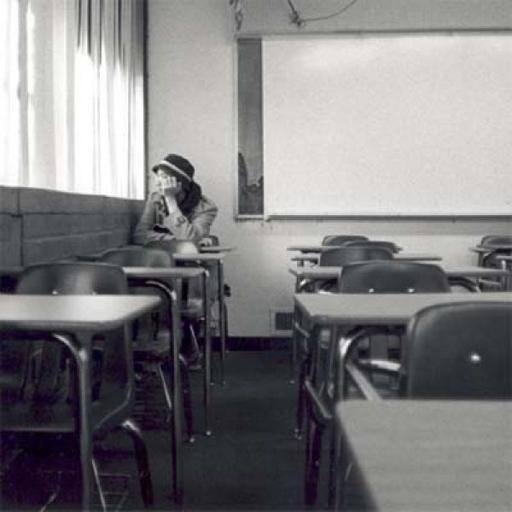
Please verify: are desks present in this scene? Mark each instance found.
[169,252,223,437]
[325,393,512,511]
[203,242,233,358]
[3,294,160,512]
[292,290,512,511]
[121,264,199,510]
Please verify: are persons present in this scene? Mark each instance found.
[132,155,218,370]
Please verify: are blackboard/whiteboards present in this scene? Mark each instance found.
[234,30,512,220]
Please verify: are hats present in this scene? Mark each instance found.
[151,153,195,185]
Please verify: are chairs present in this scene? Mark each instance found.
[160,234,225,385]
[128,241,199,442]
[288,233,510,389]
[78,245,179,458]
[398,302,509,396]
[3,262,152,512]
